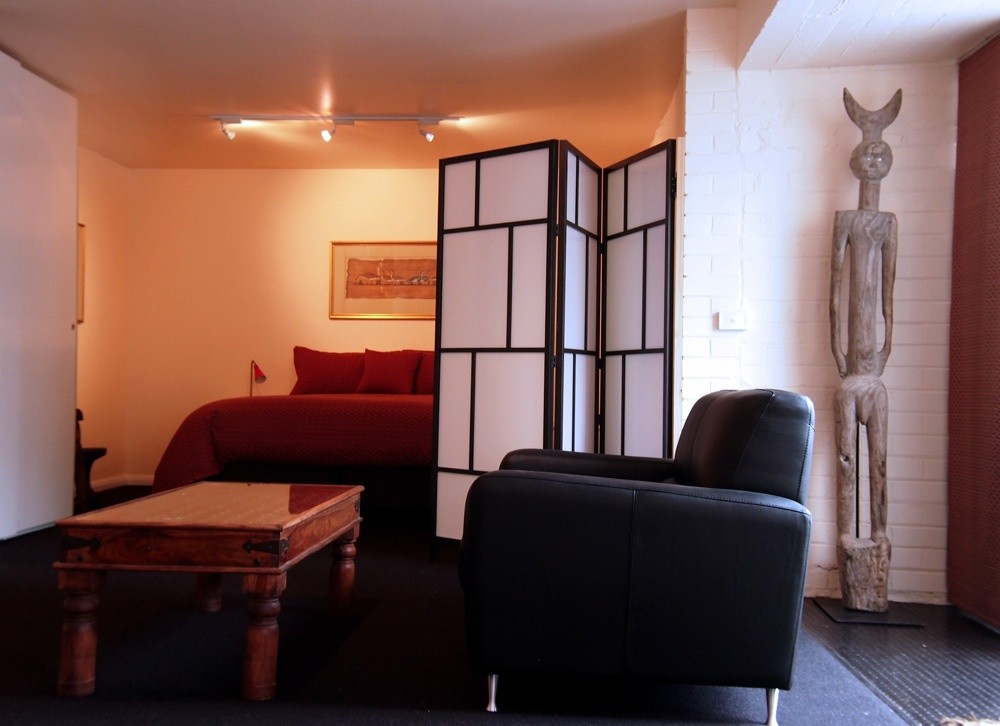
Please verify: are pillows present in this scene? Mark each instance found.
[401,349,434,393]
[290,347,365,395]
[355,349,421,394]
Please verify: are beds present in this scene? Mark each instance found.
[153,390,435,528]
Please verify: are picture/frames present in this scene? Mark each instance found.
[328,240,437,320]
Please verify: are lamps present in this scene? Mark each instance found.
[250,360,266,397]
[417,120,439,142]
[321,119,354,142]
[220,118,242,139]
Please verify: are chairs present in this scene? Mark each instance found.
[461,389,813,726]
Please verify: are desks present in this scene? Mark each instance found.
[52,481,365,700]
